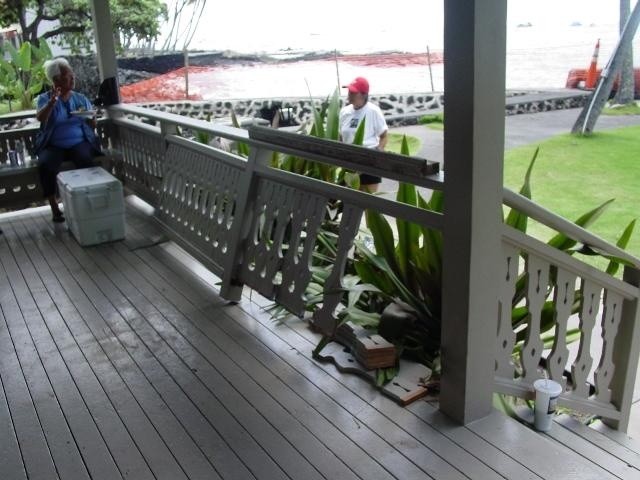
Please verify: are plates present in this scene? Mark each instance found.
[70,111,96,116]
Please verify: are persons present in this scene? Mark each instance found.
[329,76,388,198]
[32,59,103,225]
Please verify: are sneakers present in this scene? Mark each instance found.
[52,208,65,223]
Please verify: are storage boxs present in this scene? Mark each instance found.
[56,167,125,247]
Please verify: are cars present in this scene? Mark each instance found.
[567,64,639,102]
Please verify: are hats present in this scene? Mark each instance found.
[342,77,369,94]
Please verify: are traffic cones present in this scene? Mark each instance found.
[587,38,601,92]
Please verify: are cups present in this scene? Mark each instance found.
[533,378,563,432]
